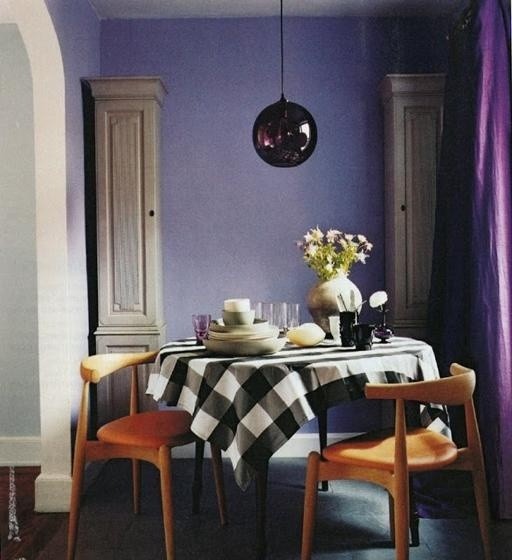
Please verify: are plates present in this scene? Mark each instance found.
[208,320,280,341]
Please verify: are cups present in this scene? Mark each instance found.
[329,311,374,352]
[254,301,299,335]
[190,313,218,345]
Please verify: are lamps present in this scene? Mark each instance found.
[251,0,316,169]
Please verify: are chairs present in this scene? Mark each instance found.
[300,361,497,560]
[64,349,230,559]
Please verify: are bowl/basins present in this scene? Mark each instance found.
[220,298,256,330]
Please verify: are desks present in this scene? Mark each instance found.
[155,334,432,560]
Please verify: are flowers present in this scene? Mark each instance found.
[292,224,375,279]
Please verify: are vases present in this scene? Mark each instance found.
[305,274,364,338]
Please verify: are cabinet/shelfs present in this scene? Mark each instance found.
[378,71,452,429]
[82,71,166,421]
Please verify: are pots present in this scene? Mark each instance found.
[203,329,292,356]
